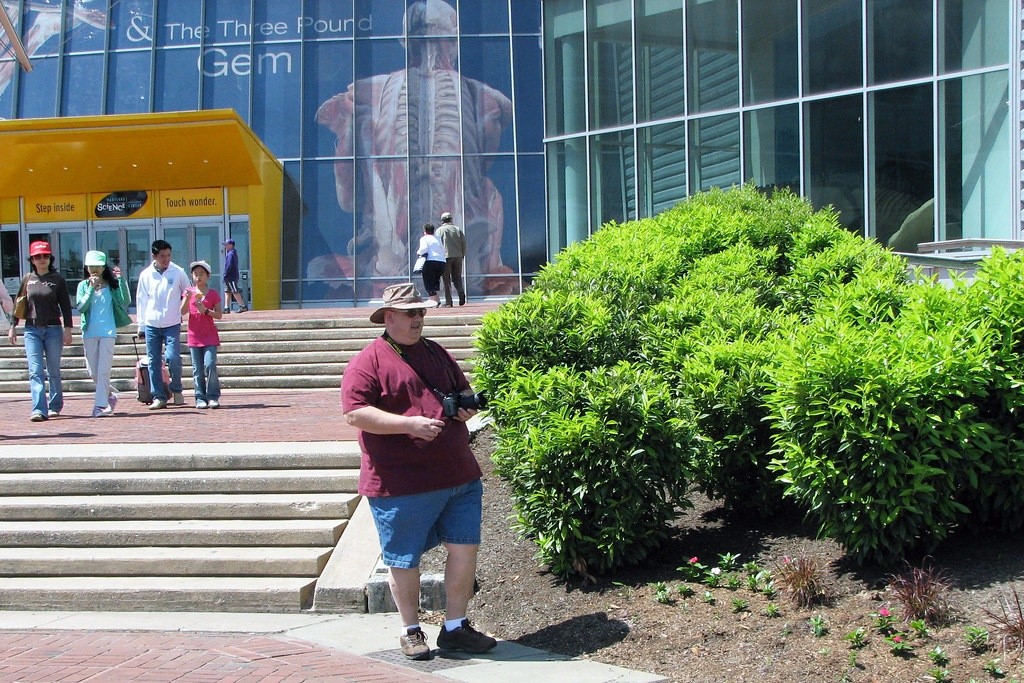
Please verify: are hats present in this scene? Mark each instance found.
[369,283,438,324]
[441,212,452,221]
[189,260,212,275]
[30,241,51,256]
[222,239,235,246]
[84,251,106,267]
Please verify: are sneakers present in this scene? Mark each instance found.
[436,618,497,654]
[400,626,429,660]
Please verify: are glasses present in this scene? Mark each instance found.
[34,254,50,260]
[385,308,427,318]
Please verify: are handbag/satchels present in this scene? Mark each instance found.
[413,253,428,274]
[13,272,30,319]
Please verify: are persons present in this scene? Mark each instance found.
[76,251,131,421]
[0,278,14,326]
[136,240,191,409]
[341,283,497,660]
[417,223,446,309]
[180,260,222,409]
[8,241,74,421]
[221,239,248,315]
[434,211,466,308]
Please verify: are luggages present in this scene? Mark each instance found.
[132,336,172,405]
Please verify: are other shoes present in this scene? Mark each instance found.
[221,308,230,314]
[236,305,248,313]
[208,399,219,408]
[103,393,118,414]
[31,414,46,421]
[442,302,453,307]
[92,409,107,417]
[48,409,59,417]
[174,391,184,405]
[436,300,441,308]
[458,293,465,306]
[196,398,207,408]
[148,397,168,409]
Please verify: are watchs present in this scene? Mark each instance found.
[204,308,210,315]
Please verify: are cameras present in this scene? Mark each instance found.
[442,390,490,417]
